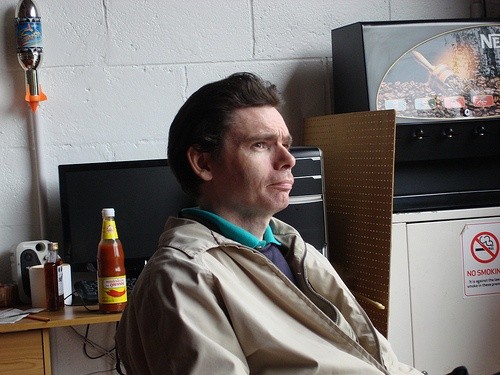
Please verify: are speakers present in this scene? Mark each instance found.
[10,240,56,309]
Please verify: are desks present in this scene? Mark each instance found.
[0,304,129,374]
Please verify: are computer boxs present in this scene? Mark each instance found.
[272,146,328,261]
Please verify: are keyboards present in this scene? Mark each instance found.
[74,277,137,303]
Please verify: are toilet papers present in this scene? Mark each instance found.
[29,263,72,308]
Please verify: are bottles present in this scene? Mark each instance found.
[95,208,128,314]
[42,241,65,312]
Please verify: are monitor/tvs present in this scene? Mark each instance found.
[58,159,200,272]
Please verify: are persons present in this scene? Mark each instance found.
[115,72,425,375]
